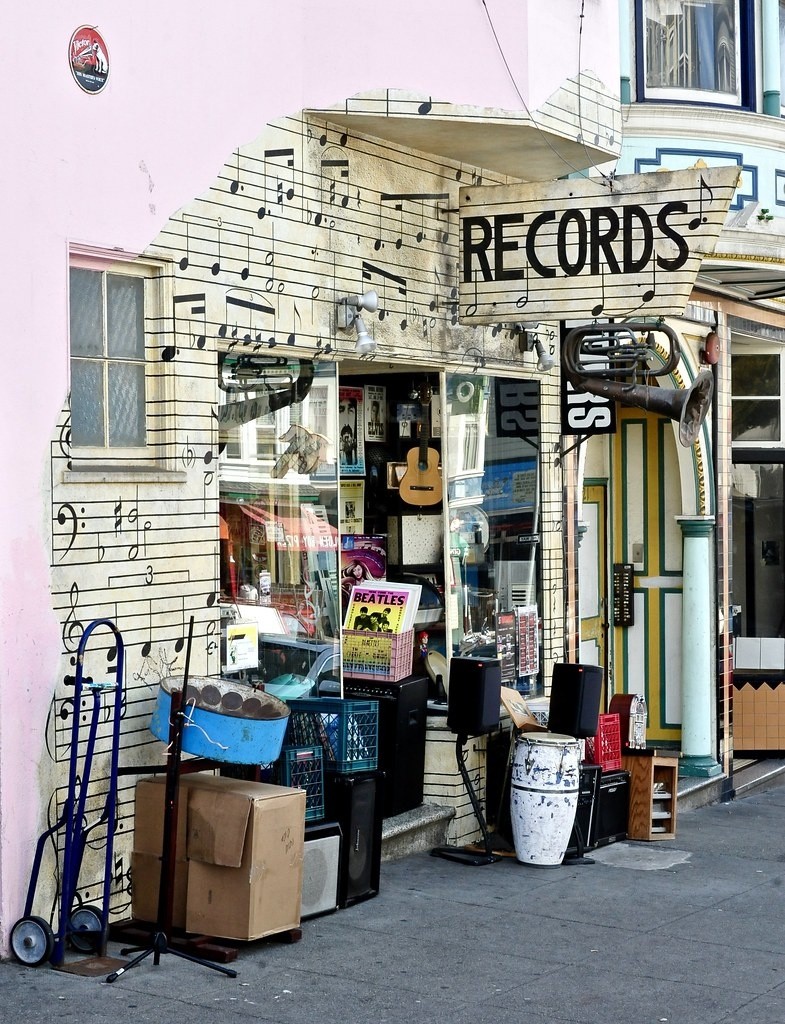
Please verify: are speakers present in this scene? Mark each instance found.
[565,764,631,856]
[548,662,604,739]
[342,676,428,818]
[446,657,502,736]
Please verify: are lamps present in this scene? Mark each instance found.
[338,290,379,354]
[534,340,555,372]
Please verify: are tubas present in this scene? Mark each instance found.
[563,321,715,449]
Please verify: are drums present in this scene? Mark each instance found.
[509,731,583,871]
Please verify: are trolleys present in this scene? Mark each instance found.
[9,618,125,967]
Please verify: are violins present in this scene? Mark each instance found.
[400,380,445,505]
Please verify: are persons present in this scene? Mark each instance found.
[368,400,383,436]
[341,560,376,599]
[340,396,357,466]
[355,607,392,634]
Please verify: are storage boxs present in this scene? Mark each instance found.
[339,626,415,682]
[286,697,381,774]
[584,713,622,774]
[384,509,446,572]
[132,772,307,942]
[219,744,325,824]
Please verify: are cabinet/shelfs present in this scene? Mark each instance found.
[619,749,684,841]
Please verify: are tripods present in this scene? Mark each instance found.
[107,616,238,988]
